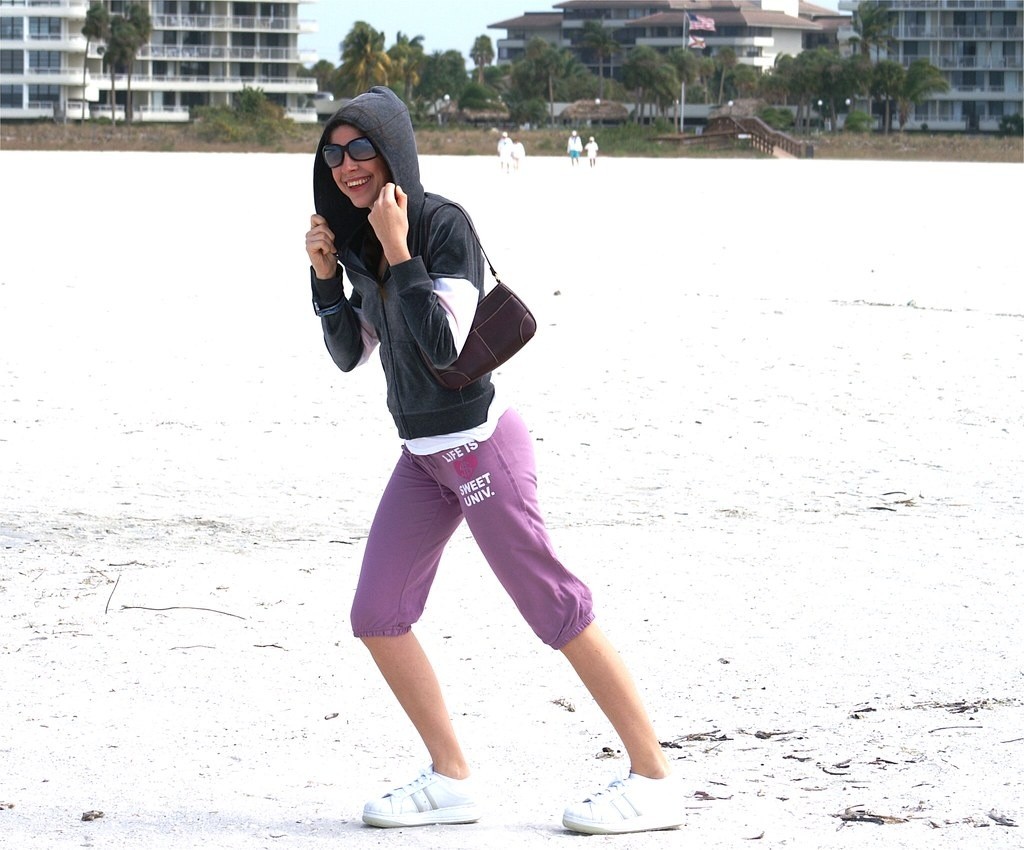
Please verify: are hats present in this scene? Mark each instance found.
[503,132,508,138]
[589,136,594,141]
[572,130,577,136]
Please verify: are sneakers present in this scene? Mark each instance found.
[363,763,481,828]
[563,773,685,833]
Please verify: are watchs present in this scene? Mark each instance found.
[311,293,347,317]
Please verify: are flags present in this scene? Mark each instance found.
[688,36,707,50]
[684,11,716,32]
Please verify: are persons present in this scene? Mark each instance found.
[306,85,683,834]
[498,130,599,175]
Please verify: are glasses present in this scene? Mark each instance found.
[321,137,378,168]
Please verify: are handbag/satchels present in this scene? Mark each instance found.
[419,202,537,390]
